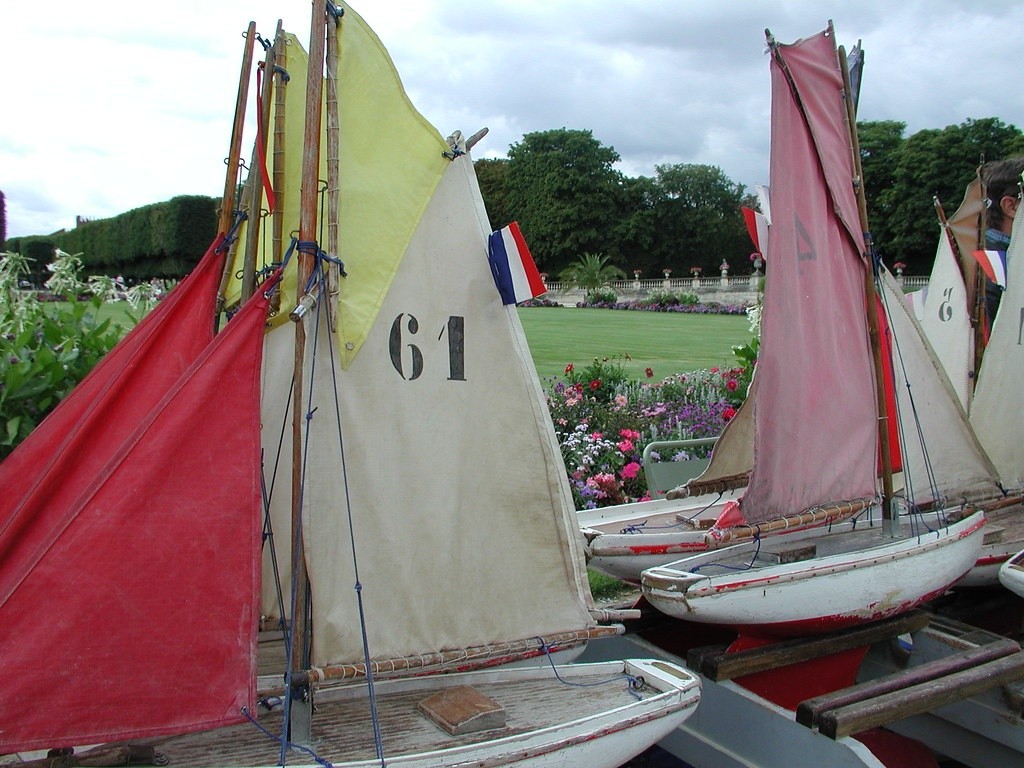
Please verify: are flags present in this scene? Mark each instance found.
[489,221,548,306]
[741,204,768,263]
[972,250,1008,289]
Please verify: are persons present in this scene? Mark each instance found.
[973,156,1024,338]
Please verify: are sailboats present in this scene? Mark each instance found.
[569,15,1024,636]
[0,1,703,768]
